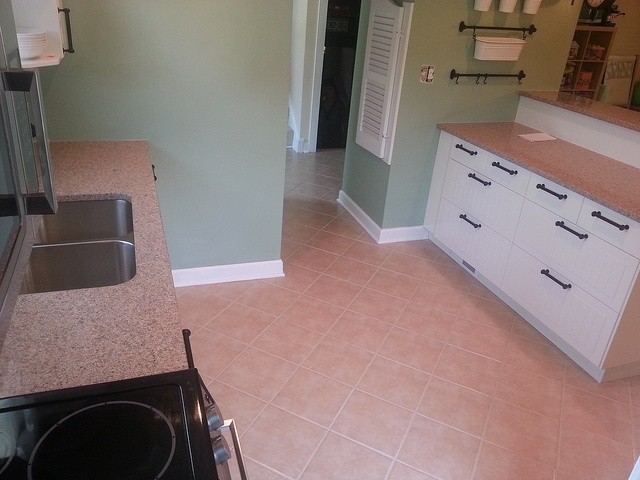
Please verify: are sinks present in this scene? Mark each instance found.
[33,193,133,244]
[17,240,136,296]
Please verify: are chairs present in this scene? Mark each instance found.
[434,135,531,288]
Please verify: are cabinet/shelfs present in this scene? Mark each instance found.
[559,26,617,99]
[499,173,639,368]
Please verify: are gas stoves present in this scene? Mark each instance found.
[0,367,246,480]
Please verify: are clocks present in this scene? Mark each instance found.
[577,0,617,27]
